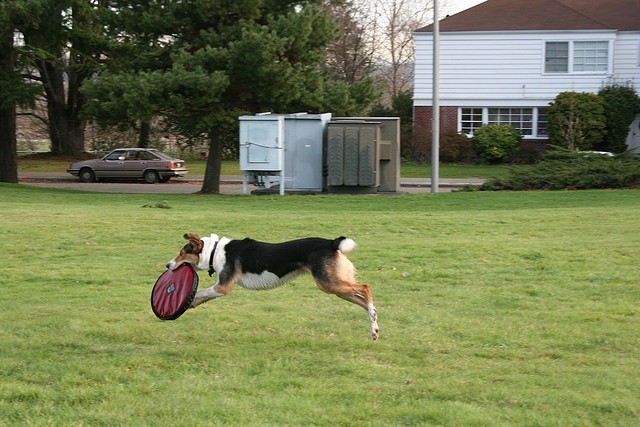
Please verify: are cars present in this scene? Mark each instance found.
[66,147,189,183]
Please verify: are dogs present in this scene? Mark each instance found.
[165,231,380,343]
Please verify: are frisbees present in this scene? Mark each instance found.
[151,262,199,320]
